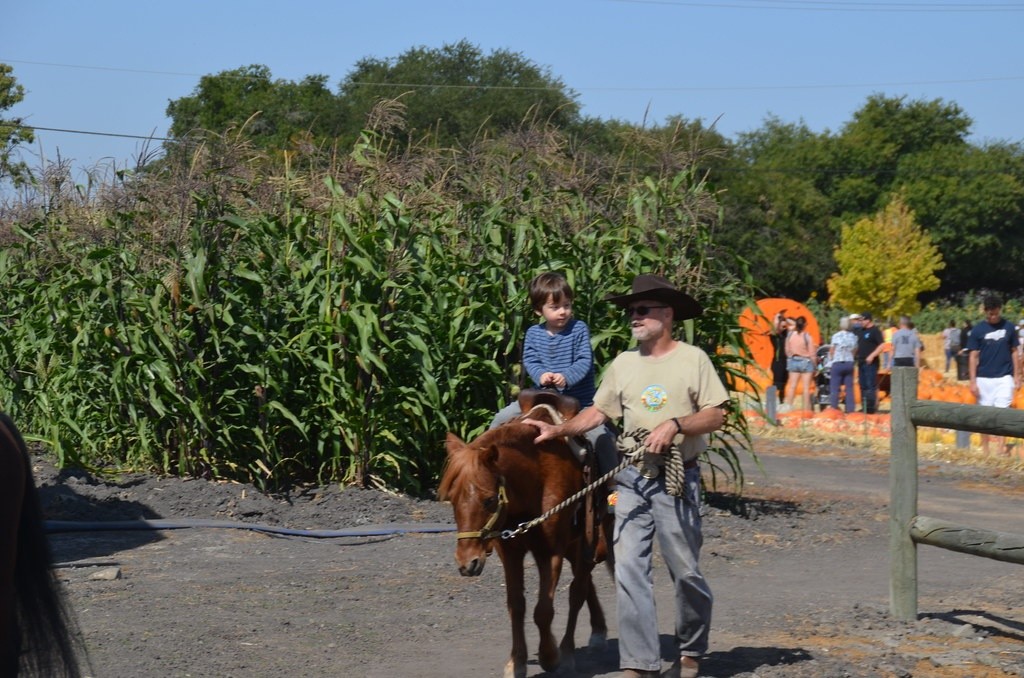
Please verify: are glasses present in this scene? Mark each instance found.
[625,302,668,316]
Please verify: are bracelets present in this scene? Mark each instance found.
[671,417,682,433]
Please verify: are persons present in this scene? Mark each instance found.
[488,273,618,514]
[966,295,1021,459]
[769,310,925,415]
[942,319,973,374]
[521,274,731,678]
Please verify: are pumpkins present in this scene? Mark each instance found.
[719,364,1024,450]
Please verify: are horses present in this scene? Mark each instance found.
[440,404,615,678]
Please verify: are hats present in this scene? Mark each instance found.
[611,274,699,320]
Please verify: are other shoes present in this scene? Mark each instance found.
[607,490,618,513]
[849,314,862,319]
[667,654,699,678]
[616,670,648,678]
